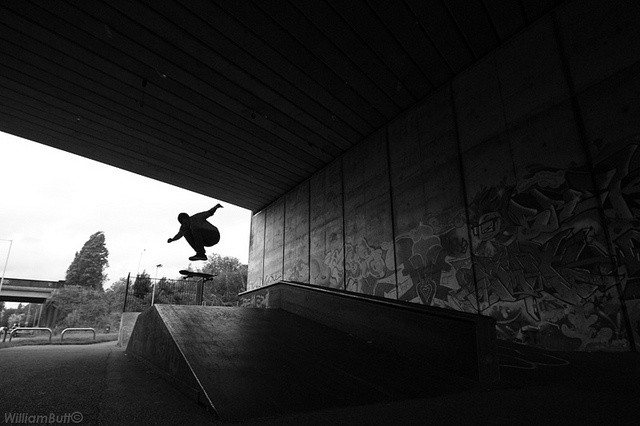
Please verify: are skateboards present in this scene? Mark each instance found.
[178,269,217,283]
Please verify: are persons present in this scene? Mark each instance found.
[166,203,225,261]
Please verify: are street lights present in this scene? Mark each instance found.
[151,264,162,307]
